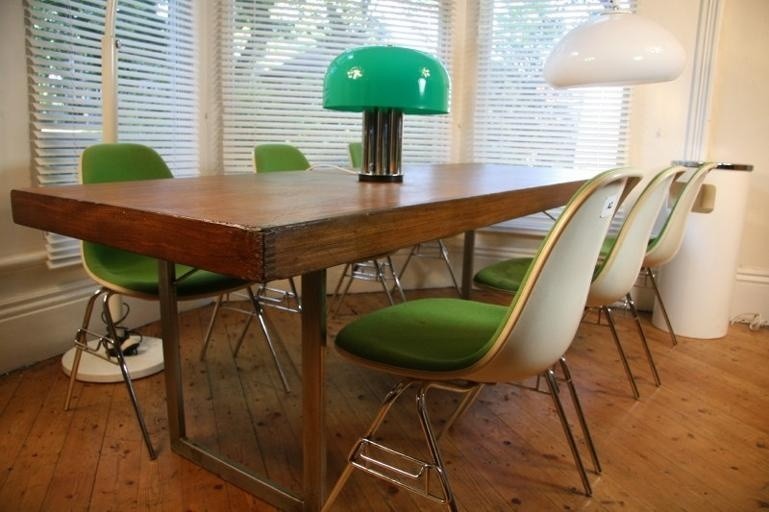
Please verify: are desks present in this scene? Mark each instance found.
[11,163,598,512]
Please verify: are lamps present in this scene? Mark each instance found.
[544,0,690,89]
[322,45,449,182]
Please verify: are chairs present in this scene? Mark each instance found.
[332,144,464,313]
[318,168,645,511]
[599,162,716,388]
[233,148,403,360]
[451,166,687,475]
[64,145,292,462]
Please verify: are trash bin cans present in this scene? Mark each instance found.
[650,158,754,340]
